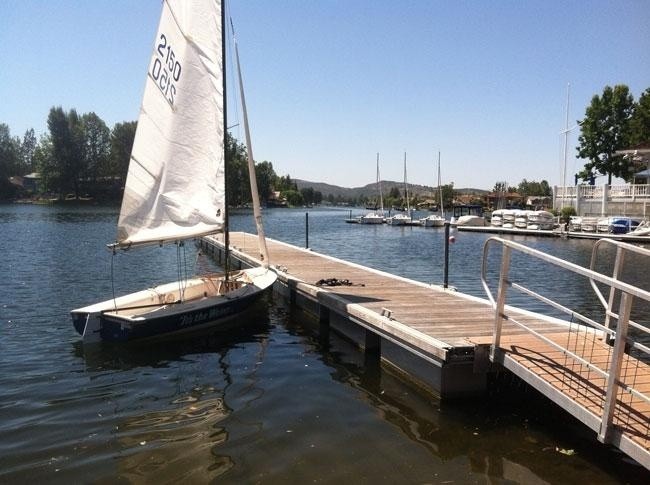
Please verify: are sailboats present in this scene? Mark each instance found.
[69,2,278,348]
[357,150,448,228]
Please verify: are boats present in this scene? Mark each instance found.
[569,217,640,234]
